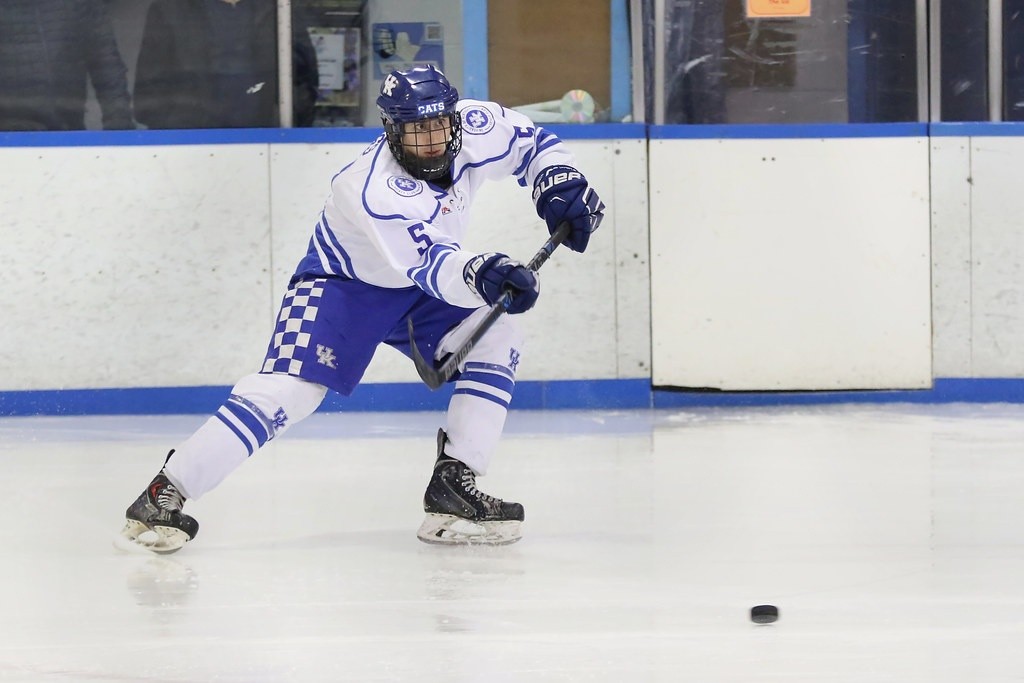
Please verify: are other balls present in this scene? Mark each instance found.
[749,604,779,624]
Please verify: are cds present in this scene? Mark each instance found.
[560,89,594,122]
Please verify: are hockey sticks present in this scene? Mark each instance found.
[404,219,572,392]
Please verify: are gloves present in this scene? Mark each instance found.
[533,165,605,253]
[463,252,540,315]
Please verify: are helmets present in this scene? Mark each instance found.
[376,63,462,181]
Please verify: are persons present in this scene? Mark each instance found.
[115,64,606,554]
[0,0,319,132]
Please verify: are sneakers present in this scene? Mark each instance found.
[417,427,524,545]
[114,449,199,555]
